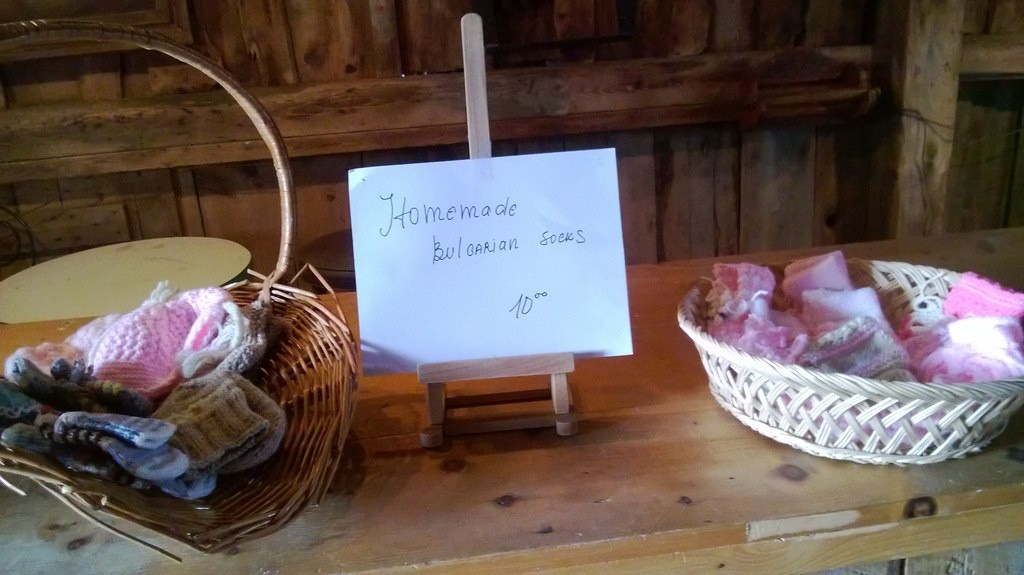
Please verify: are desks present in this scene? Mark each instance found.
[0,223,1024,575]
[0,235,256,329]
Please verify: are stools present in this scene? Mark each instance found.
[298,226,357,295]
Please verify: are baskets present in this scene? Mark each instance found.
[676,256,1024,469]
[0,19,364,563]
